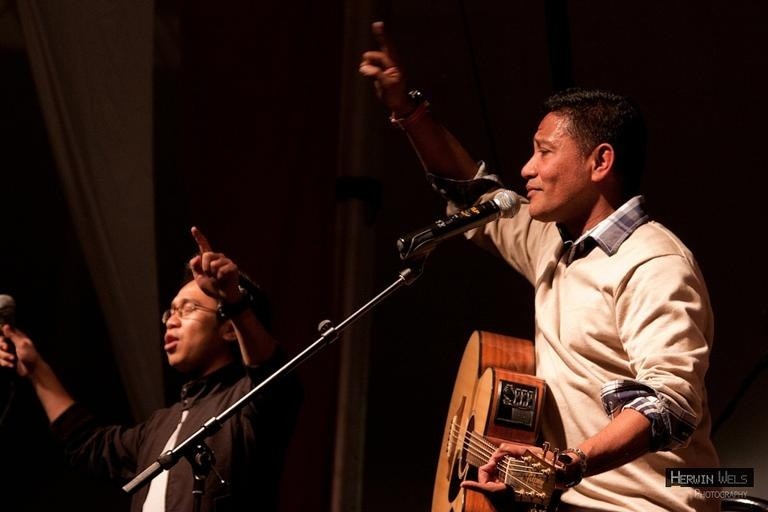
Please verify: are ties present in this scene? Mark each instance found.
[141,410,190,512]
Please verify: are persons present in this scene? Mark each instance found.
[0,226,303,512]
[356,20,725,512]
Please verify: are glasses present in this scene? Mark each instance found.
[159,303,218,325]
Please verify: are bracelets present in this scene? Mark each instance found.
[387,88,436,131]
[218,286,255,321]
[562,447,589,475]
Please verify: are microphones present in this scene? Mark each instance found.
[396,190,521,257]
[0,294,18,382]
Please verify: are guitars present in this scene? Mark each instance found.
[429,332,567,512]
